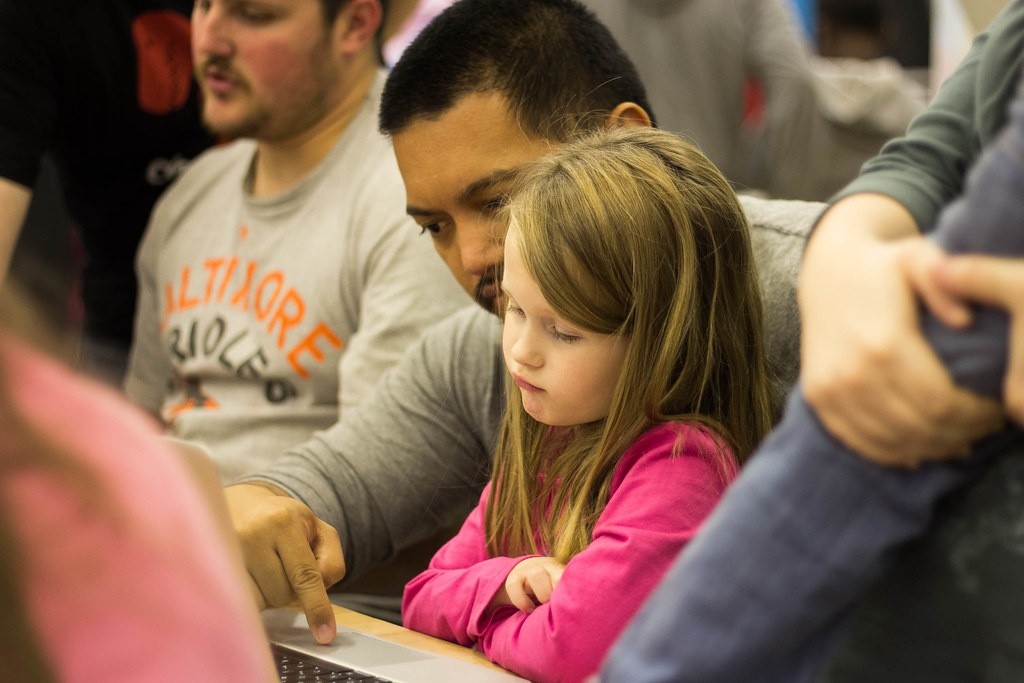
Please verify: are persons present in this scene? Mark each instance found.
[600,0,1024,683]
[222,0,658,644]
[0,0,220,387]
[0,282,280,683]
[125,0,476,482]
[579,0,816,199]
[401,127,773,683]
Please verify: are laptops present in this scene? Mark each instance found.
[157,435,531,683]
